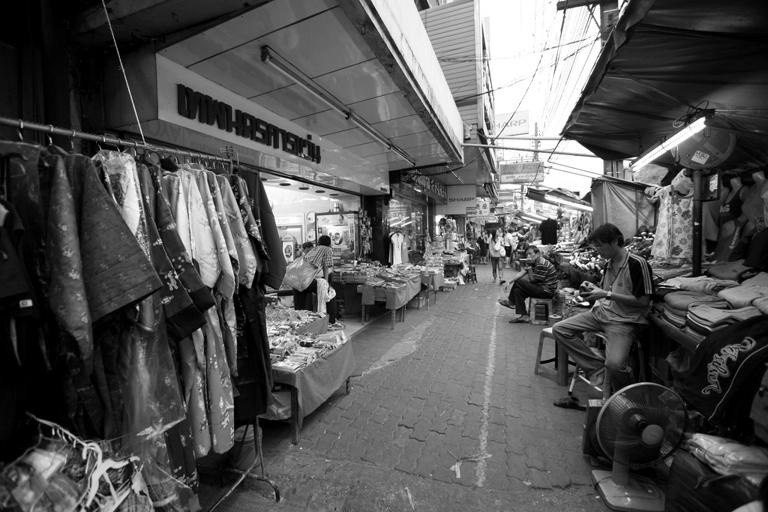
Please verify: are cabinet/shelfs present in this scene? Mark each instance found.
[314,211,361,260]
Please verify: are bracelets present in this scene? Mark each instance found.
[606,291,611,301]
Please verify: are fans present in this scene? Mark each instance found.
[590,382,690,512]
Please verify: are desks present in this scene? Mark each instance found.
[265,302,350,445]
[356,269,421,329]
[405,265,438,311]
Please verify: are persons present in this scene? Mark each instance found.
[301,242,313,255]
[714,175,749,262]
[553,223,655,394]
[497,246,558,323]
[740,171,768,272]
[488,234,506,286]
[478,227,531,268]
[297,235,337,324]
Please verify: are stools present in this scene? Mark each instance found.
[568,330,650,406]
[466,273,477,283]
[535,329,590,386]
[468,265,475,272]
[529,297,554,325]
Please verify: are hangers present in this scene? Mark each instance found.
[7,118,240,179]
[1,419,144,512]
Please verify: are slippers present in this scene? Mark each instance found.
[508,318,528,323]
[553,396,587,411]
[498,299,515,309]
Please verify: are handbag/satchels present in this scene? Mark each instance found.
[281,256,318,292]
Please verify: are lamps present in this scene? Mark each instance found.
[348,110,391,150]
[628,115,710,174]
[544,193,593,212]
[389,141,415,169]
[259,44,351,122]
